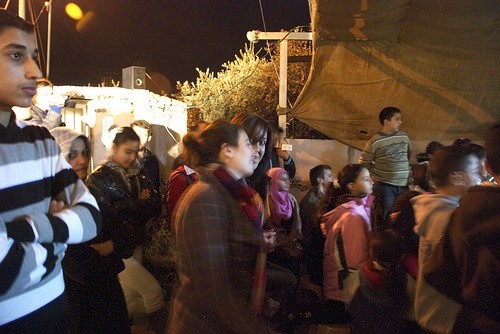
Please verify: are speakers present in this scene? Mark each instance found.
[121,66,146,89]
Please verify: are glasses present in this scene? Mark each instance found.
[249,137,268,147]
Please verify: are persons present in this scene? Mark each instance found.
[0,10,105,334]
[86,128,162,334]
[171,118,285,334]
[267,168,309,271]
[319,164,375,312]
[359,107,412,236]
[130,120,159,196]
[51,128,130,334]
[143,122,209,334]
[348,123,500,334]
[301,164,333,283]
[232,113,297,334]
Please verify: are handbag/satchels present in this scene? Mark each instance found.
[334,211,387,302]
